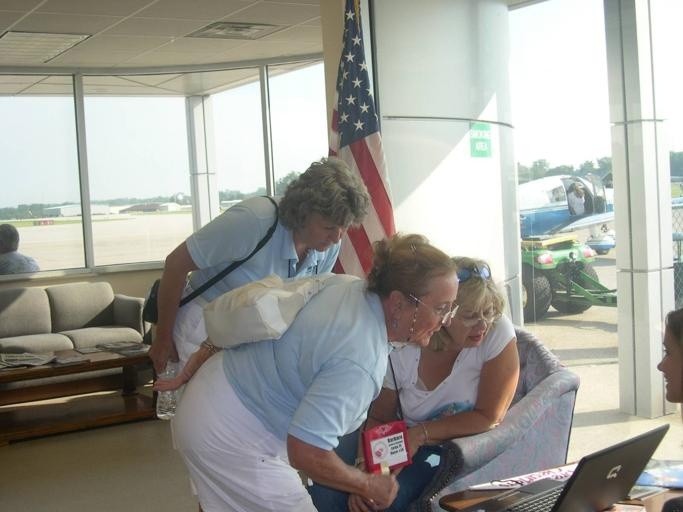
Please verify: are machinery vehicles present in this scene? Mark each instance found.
[521,232,683,323]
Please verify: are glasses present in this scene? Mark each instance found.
[457,312,501,329]
[457,265,491,284]
[408,294,459,325]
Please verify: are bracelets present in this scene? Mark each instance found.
[200,340,216,355]
[419,422,428,441]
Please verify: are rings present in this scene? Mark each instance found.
[368,498,375,504]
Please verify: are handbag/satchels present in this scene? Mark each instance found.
[142,194,280,323]
[203,274,363,348]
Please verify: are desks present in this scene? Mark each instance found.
[439,460,683,512]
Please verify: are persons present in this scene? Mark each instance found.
[153,233,459,512]
[144,155,368,512]
[642,306,683,511]
[0,222,42,273]
[307,255,520,512]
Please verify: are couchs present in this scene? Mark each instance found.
[0,283,152,354]
[405,324,580,512]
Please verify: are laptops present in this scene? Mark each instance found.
[437,423,673,511]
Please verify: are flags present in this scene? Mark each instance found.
[324,0,397,277]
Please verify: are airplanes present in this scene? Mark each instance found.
[517,171,683,255]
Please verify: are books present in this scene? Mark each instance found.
[626,485,674,501]
